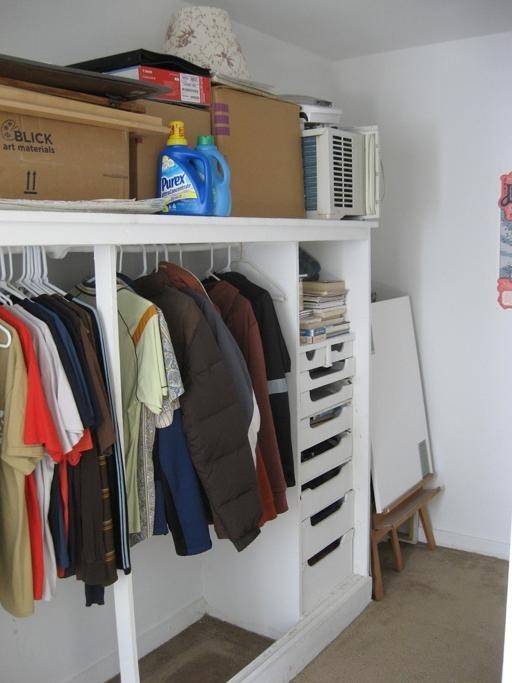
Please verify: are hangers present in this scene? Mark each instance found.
[1,245,284,346]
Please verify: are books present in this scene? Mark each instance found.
[299,272,350,346]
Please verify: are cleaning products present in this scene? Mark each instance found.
[189,134,231,217]
[158,121,213,215]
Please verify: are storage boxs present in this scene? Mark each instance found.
[0,67,307,219]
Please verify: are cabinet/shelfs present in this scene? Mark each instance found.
[205,241,358,641]
